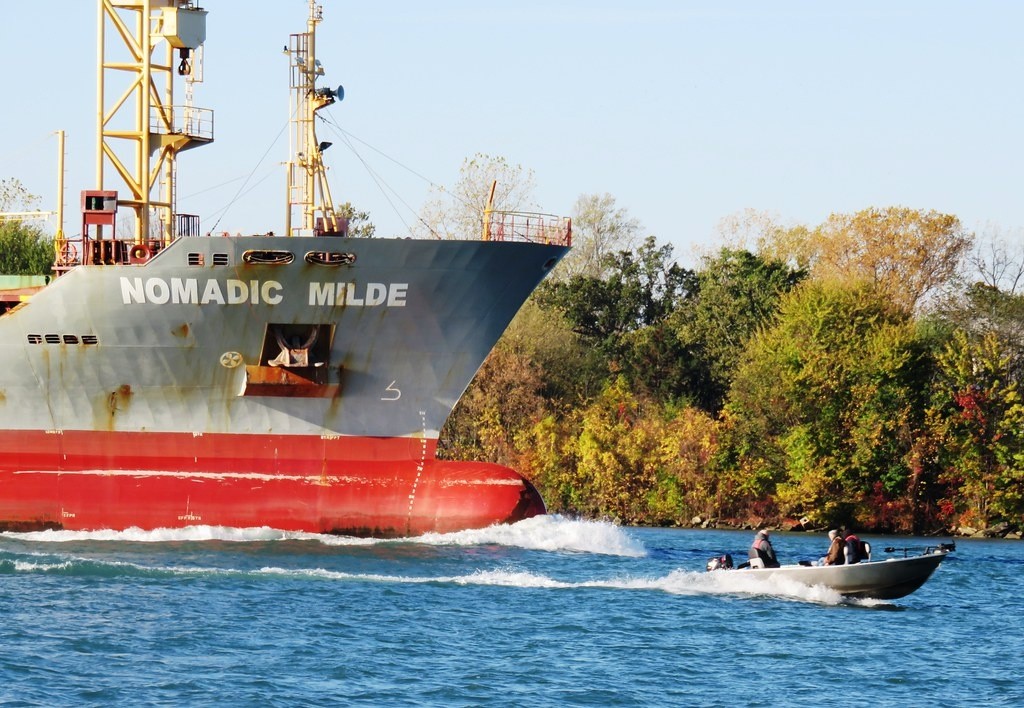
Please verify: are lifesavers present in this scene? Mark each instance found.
[129,245,151,263]
[61,244,77,263]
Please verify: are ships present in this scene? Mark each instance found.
[0,210,577,539]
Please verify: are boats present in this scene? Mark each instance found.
[694,536,958,601]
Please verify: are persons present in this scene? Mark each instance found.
[841,530,860,564]
[752,530,780,568]
[818,530,845,566]
